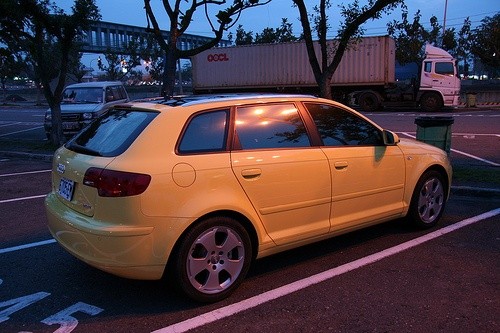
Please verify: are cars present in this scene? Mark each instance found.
[43,92,453,305]
[44,81,130,144]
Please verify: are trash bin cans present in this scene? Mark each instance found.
[414,116,454,157]
[465,92,478,107]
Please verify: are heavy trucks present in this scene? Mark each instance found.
[189,34,462,113]
